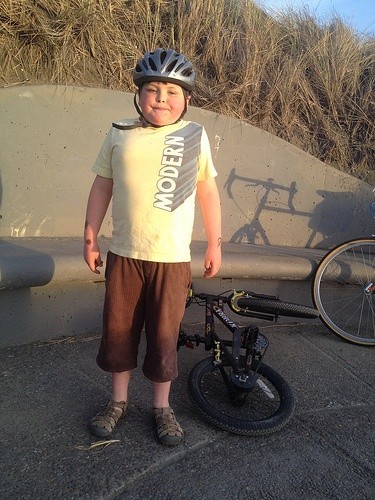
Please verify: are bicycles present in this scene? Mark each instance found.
[311,234,375,348]
[175,281,321,437]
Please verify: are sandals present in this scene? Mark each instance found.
[88,399,127,437]
[151,406,183,446]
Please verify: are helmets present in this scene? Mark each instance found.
[133,48,195,106]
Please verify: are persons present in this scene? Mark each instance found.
[84,48,222,445]
